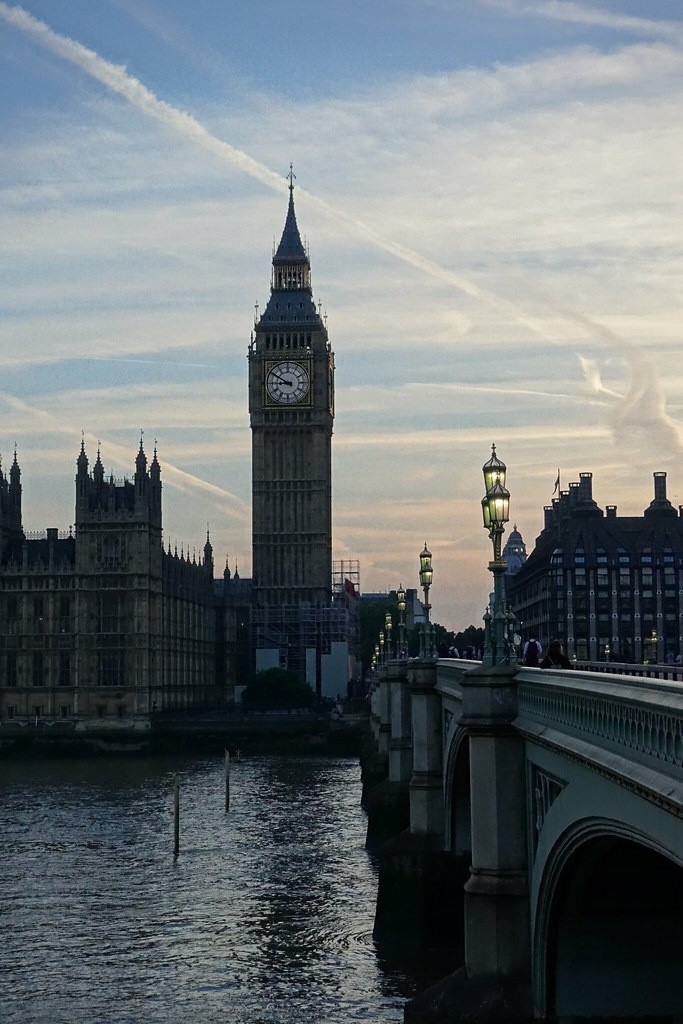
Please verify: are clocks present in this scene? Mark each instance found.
[264,361,310,405]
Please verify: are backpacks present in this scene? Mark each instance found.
[525,640,538,660]
[465,646,473,658]
[548,657,562,669]
[450,649,458,658]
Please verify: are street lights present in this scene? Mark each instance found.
[384,609,394,662]
[370,628,387,673]
[419,549,440,661]
[480,444,516,665]
[396,583,409,660]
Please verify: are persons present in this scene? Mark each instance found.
[523,633,542,667]
[437,640,484,661]
[538,641,574,670]
